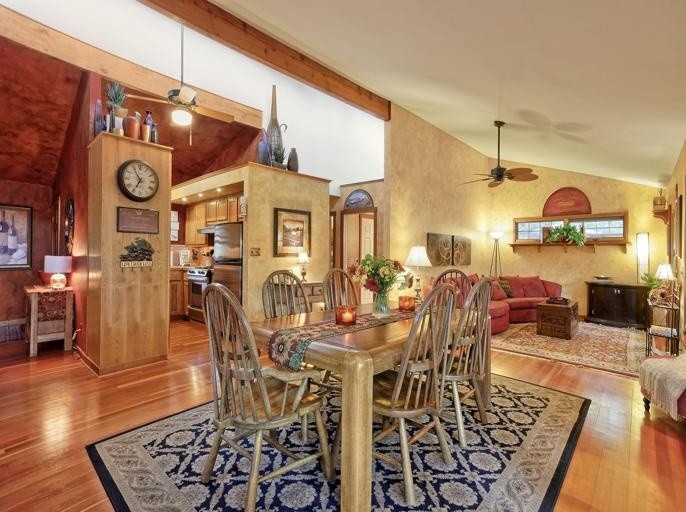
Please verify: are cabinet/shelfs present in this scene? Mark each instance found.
[169,192,245,335]
[645,282,681,358]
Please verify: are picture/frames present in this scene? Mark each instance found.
[0,204,32,270]
[273,206,311,258]
[117,206,160,234]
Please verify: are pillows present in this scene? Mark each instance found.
[432,273,548,307]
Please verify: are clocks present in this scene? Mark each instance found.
[117,158,160,202]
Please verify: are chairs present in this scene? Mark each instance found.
[435,268,473,307]
[320,265,366,381]
[391,279,490,462]
[258,266,311,321]
[330,283,458,508]
[200,282,334,510]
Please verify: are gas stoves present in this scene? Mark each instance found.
[186,266,213,276]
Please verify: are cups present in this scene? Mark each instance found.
[398,295,415,312]
[205,257,213,267]
[335,305,355,326]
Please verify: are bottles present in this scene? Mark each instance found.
[104,111,161,146]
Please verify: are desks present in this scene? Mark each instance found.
[583,280,653,330]
[23,283,74,358]
[247,294,490,511]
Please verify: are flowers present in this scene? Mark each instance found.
[350,253,410,292]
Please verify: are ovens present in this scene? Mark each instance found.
[187,269,216,307]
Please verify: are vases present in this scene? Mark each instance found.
[287,147,299,172]
[370,294,394,318]
[123,115,140,140]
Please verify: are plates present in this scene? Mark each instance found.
[584,280,614,285]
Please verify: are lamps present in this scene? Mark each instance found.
[170,105,192,126]
[489,231,502,277]
[44,255,73,289]
[403,246,432,305]
[296,252,309,282]
[654,264,677,284]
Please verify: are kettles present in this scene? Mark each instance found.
[179,250,191,267]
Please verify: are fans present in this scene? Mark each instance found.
[121,22,234,125]
[463,119,539,188]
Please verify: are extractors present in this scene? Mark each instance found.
[196,227,215,235]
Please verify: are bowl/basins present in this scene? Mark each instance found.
[593,274,612,279]
[36,270,53,285]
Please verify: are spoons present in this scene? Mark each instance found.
[206,252,209,256]
[210,249,214,257]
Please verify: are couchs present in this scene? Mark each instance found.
[424,279,562,333]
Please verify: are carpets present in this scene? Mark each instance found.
[490,318,657,377]
[85,363,591,511]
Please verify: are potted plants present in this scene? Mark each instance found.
[103,80,128,118]
[544,218,587,248]
[653,187,666,211]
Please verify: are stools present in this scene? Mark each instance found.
[639,353,686,421]
